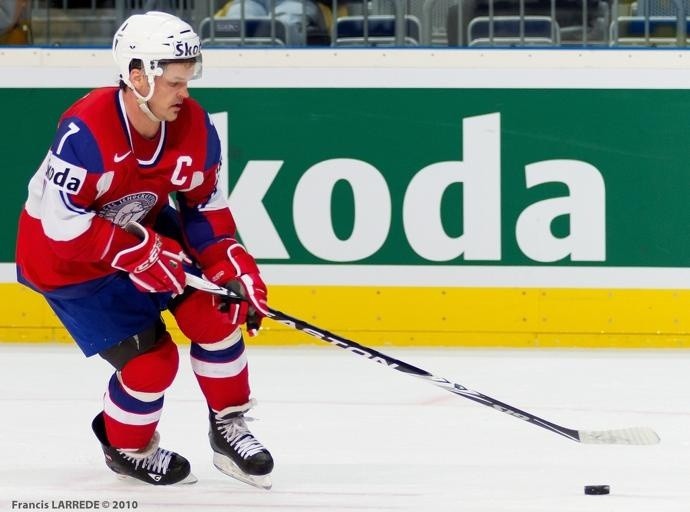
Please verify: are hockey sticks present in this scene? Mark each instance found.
[181,263,662,445]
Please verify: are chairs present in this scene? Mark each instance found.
[468,38,556,47]
[609,16,690,42]
[199,17,290,47]
[609,37,690,47]
[199,37,287,46]
[331,15,422,46]
[468,17,561,47]
[331,37,418,46]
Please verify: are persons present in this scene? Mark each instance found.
[16,9,275,486]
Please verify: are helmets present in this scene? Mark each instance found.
[113,11,202,83]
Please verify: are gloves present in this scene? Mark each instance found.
[196,238,267,336]
[111,221,192,295]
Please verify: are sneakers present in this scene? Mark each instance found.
[92,433,190,485]
[209,398,273,475]
